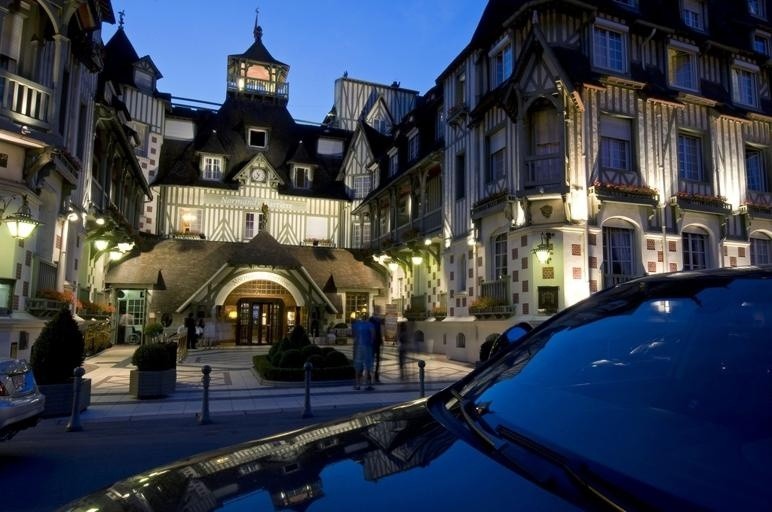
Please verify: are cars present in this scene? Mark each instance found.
[60,263,771,509]
[0,355,46,439]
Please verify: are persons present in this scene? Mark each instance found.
[393,320,412,382]
[351,312,375,392]
[174,323,186,338]
[194,318,204,335]
[185,311,197,351]
[366,304,384,383]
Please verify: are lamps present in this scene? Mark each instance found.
[533,231,552,266]
[0,192,43,243]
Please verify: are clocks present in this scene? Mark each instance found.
[248,165,271,184]
